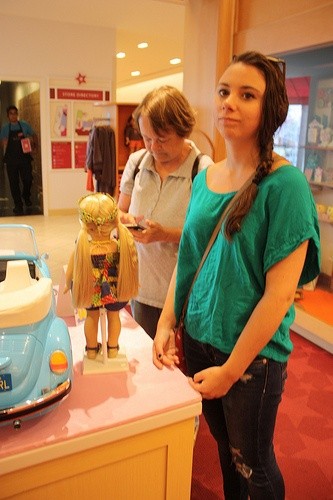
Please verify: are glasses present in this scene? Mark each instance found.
[267,55,286,84]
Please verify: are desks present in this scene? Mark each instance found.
[0,284,202,500]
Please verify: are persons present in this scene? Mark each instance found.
[123,115,144,153]
[64,193,138,359]
[0,104,36,212]
[153,51,321,500]
[118,86,215,339]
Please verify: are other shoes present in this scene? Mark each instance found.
[14,206,22,211]
[22,194,31,205]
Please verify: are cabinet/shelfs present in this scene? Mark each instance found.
[303,145,333,226]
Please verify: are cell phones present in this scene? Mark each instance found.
[125,224,146,229]
